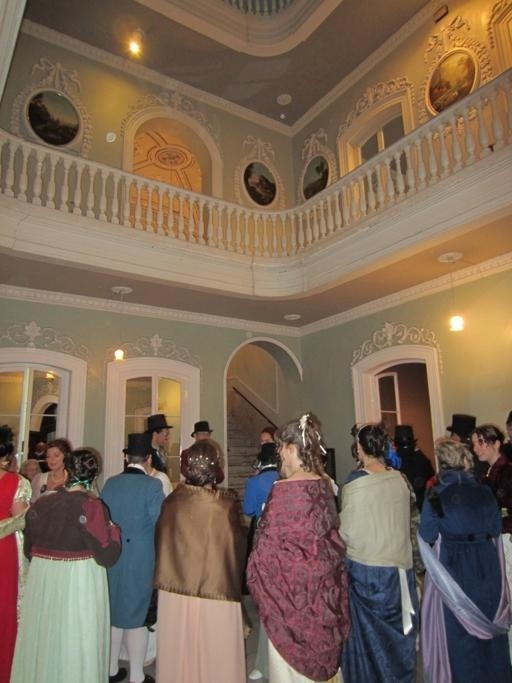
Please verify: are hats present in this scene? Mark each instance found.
[447,414,477,432]
[258,443,281,462]
[191,421,213,437]
[122,433,157,457]
[391,425,418,444]
[144,414,174,433]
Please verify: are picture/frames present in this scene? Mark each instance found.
[297,128,334,205]
[417,15,492,143]
[234,135,285,211]
[9,57,92,163]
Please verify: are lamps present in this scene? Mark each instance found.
[111,286,133,360]
[438,251,466,331]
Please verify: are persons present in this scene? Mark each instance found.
[2,411,512,683]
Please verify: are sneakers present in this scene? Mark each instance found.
[248,668,264,680]
[109,667,127,683]
[141,673,155,683]
